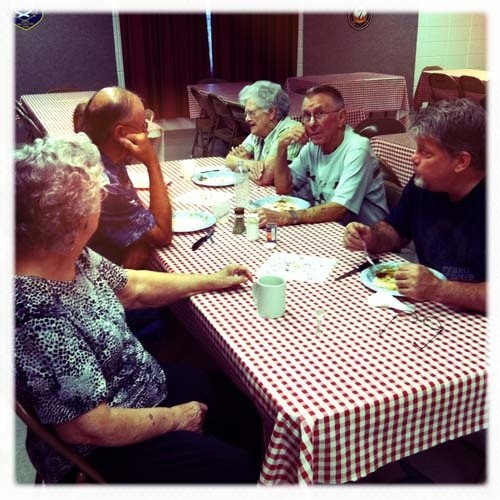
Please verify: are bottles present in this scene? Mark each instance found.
[247,211,258,241]
[234,161,249,207]
[232,207,246,234]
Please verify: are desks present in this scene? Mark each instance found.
[424,68,486,80]
[124,161,485,485]
[287,72,410,123]
[21,90,166,164]
[371,133,416,186]
[187,86,309,126]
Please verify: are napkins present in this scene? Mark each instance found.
[172,189,235,204]
[254,254,340,283]
[364,289,416,315]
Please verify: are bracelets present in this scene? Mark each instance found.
[289,210,298,226]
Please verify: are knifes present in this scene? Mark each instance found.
[192,229,215,251]
[334,257,380,282]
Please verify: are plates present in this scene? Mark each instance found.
[128,173,171,189]
[191,170,245,187]
[360,262,448,297]
[250,195,310,211]
[172,210,217,232]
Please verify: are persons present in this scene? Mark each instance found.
[345,97,487,316]
[259,85,389,228]
[225,80,303,185]
[15,132,263,484]
[77,86,178,349]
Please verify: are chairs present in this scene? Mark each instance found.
[15,99,47,141]
[15,398,106,485]
[188,87,252,159]
[420,66,486,107]
[354,117,407,138]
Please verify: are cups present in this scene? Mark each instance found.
[252,276,285,319]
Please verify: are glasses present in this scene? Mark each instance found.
[98,187,109,202]
[116,121,148,133]
[302,108,342,121]
[242,107,261,119]
